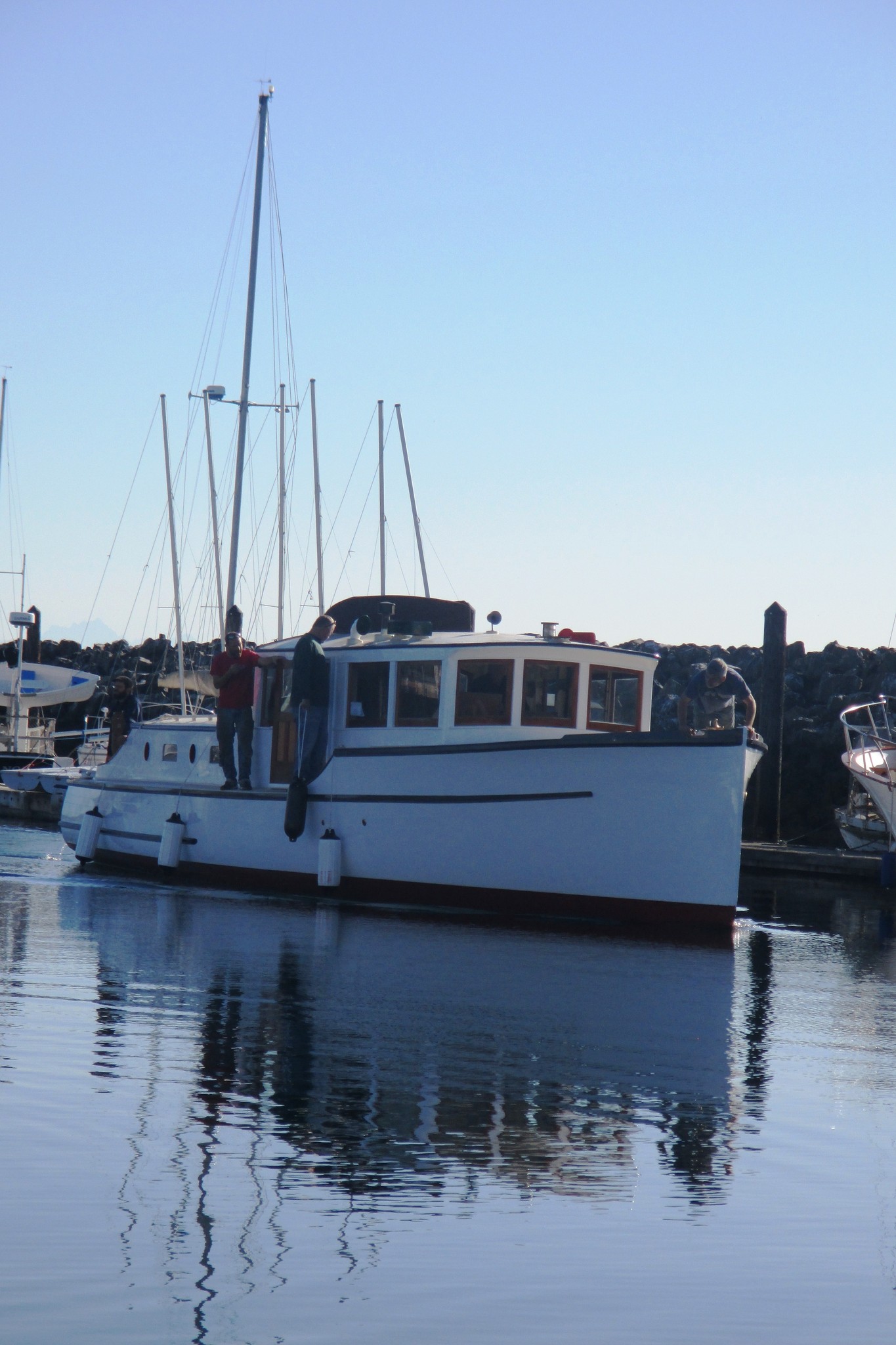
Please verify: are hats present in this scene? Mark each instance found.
[116,677,134,689]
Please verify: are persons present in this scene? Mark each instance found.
[209,632,289,790]
[291,616,337,787]
[106,676,139,764]
[678,658,757,739]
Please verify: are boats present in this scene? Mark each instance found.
[834,693,896,856]
[0,362,218,795]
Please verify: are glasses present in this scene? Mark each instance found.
[326,620,337,627]
[113,685,125,689]
[225,633,241,642]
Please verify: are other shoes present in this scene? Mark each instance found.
[239,778,252,790]
[220,778,238,790]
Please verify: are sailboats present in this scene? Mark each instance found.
[57,74,772,936]
